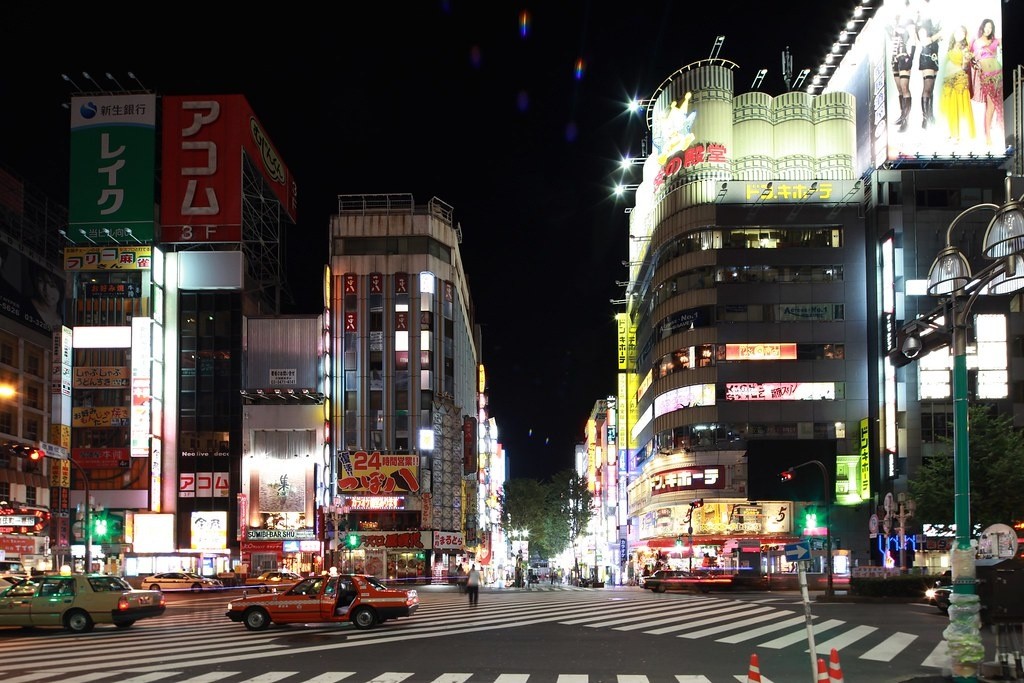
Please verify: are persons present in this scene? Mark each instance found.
[456,563,485,608]
[643,562,672,590]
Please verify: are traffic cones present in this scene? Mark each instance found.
[746,653,761,683]
[828,647,846,683]
[817,657,831,683]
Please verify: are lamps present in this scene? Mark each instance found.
[806,0,873,95]
[615,280,652,285]
[629,235,651,241]
[609,298,636,304]
[826,181,861,220]
[750,69,768,88]
[713,183,728,205]
[786,181,818,221]
[708,35,725,60]
[621,260,652,267]
[749,181,773,209]
[792,69,811,90]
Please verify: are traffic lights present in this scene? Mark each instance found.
[6,443,47,461]
[804,512,818,531]
[779,471,793,483]
[91,513,110,545]
[688,499,704,508]
[347,534,360,550]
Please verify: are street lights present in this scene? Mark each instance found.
[760,543,779,591]
[881,189,1024,683]
[513,528,529,588]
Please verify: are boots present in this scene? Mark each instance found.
[929,95,935,124]
[894,95,903,125]
[897,97,911,132]
[921,97,928,129]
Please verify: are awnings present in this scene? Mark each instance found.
[681,533,800,545]
[629,539,675,548]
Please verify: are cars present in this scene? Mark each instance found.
[638,568,731,595]
[923,584,954,615]
[244,570,304,595]
[0,561,27,581]
[0,575,166,633]
[140,571,225,594]
[224,573,420,629]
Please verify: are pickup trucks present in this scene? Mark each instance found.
[532,562,566,578]
